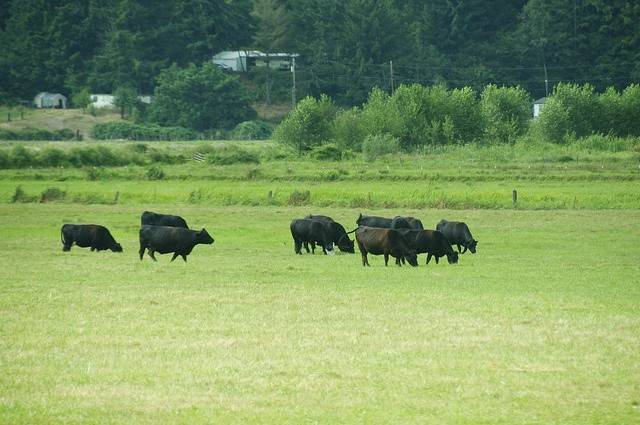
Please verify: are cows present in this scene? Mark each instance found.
[401,227,459,265]
[138,225,215,262]
[391,215,414,264]
[60,223,123,253]
[289,218,335,256]
[404,217,424,230]
[356,213,393,228]
[436,219,478,254]
[337,225,419,267]
[298,213,355,255]
[140,211,189,228]
